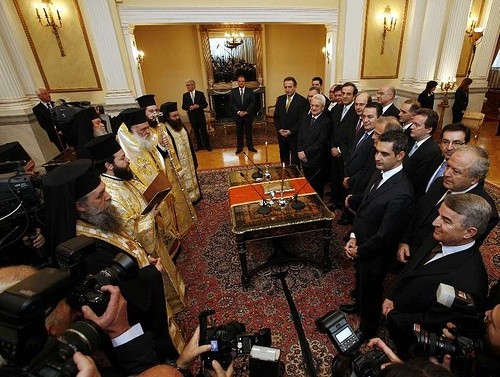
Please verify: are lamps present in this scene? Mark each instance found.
[321,46,329,61]
[136,50,145,68]
[224,31,243,49]
[36,0,66,57]
[467,15,485,52]
[381,5,397,55]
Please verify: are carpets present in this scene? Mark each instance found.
[174,161,500,377]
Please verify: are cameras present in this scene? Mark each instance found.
[318,310,391,377]
[57,236,135,319]
[0,267,104,377]
[411,282,486,361]
[198,311,285,377]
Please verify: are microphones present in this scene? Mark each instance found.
[240,172,272,214]
[292,168,321,209]
[243,151,263,179]
[285,152,313,176]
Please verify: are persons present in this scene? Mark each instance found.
[418,81,438,109]
[273,76,500,377]
[181,80,213,152]
[230,75,257,154]
[496,101,500,136]
[211,55,256,82]
[452,78,472,124]
[0,87,235,377]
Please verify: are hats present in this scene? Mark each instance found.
[70,94,179,159]
[45,158,101,202]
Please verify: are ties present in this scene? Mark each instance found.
[310,118,315,127]
[285,95,291,114]
[240,88,244,105]
[402,126,405,133]
[349,133,368,161]
[47,103,51,109]
[340,107,347,122]
[427,191,452,219]
[191,92,194,104]
[367,173,383,198]
[408,143,418,157]
[422,243,442,265]
[354,118,363,137]
[431,162,448,185]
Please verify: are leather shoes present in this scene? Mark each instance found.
[326,191,352,241]
[353,328,369,344]
[340,304,357,314]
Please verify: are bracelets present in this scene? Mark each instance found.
[171,361,188,376]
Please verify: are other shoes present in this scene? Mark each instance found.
[196,147,202,150]
[235,148,242,154]
[248,146,257,153]
[206,146,212,151]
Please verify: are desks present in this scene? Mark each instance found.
[227,164,335,289]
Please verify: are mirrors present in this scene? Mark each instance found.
[196,23,265,89]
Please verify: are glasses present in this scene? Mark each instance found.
[441,139,467,149]
[484,310,496,331]
